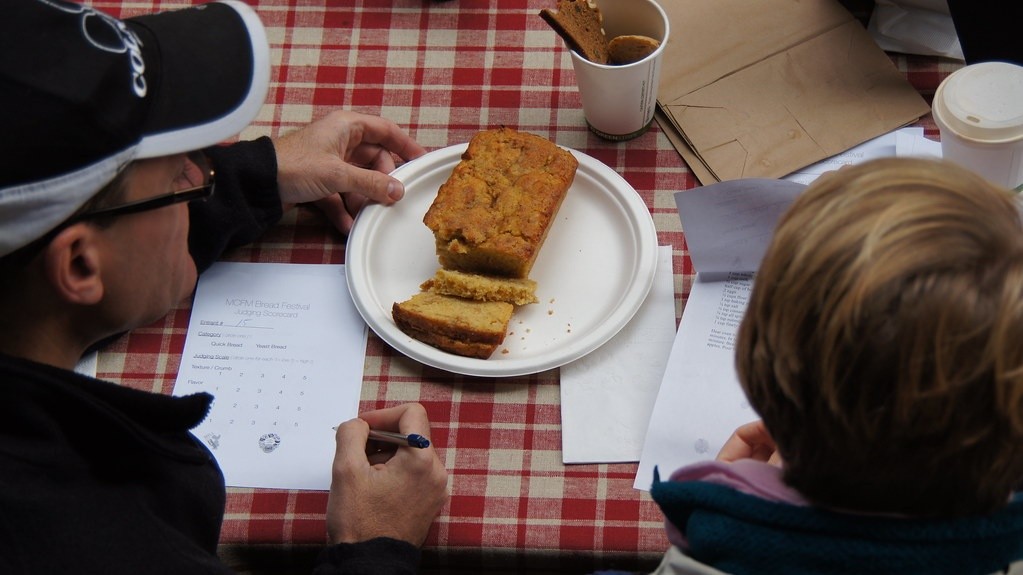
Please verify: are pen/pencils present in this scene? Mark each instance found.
[333,425,430,449]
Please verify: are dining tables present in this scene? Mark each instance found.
[0,0,978,556]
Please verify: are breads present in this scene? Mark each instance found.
[391,0,660,358]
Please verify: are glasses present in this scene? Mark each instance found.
[10,149,215,272]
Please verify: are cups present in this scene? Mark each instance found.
[563,0,669,141]
[933,62,1023,204]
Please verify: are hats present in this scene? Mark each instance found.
[0,0,271,263]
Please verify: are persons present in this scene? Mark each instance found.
[0,1,449,575]
[650,156,1023,575]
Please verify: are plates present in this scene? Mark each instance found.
[344,143,658,377]
